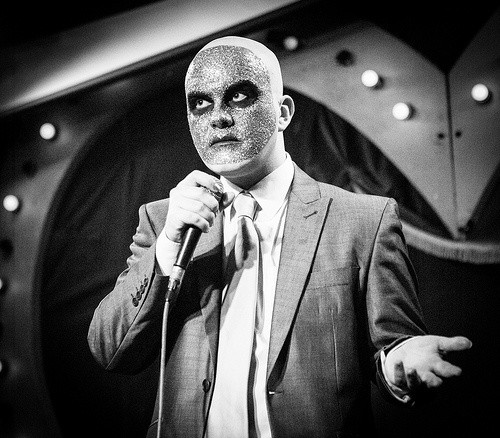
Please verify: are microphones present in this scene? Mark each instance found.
[163,175,224,303]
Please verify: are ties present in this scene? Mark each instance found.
[207,193,259,438]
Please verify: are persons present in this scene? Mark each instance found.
[86,34,472,438]
